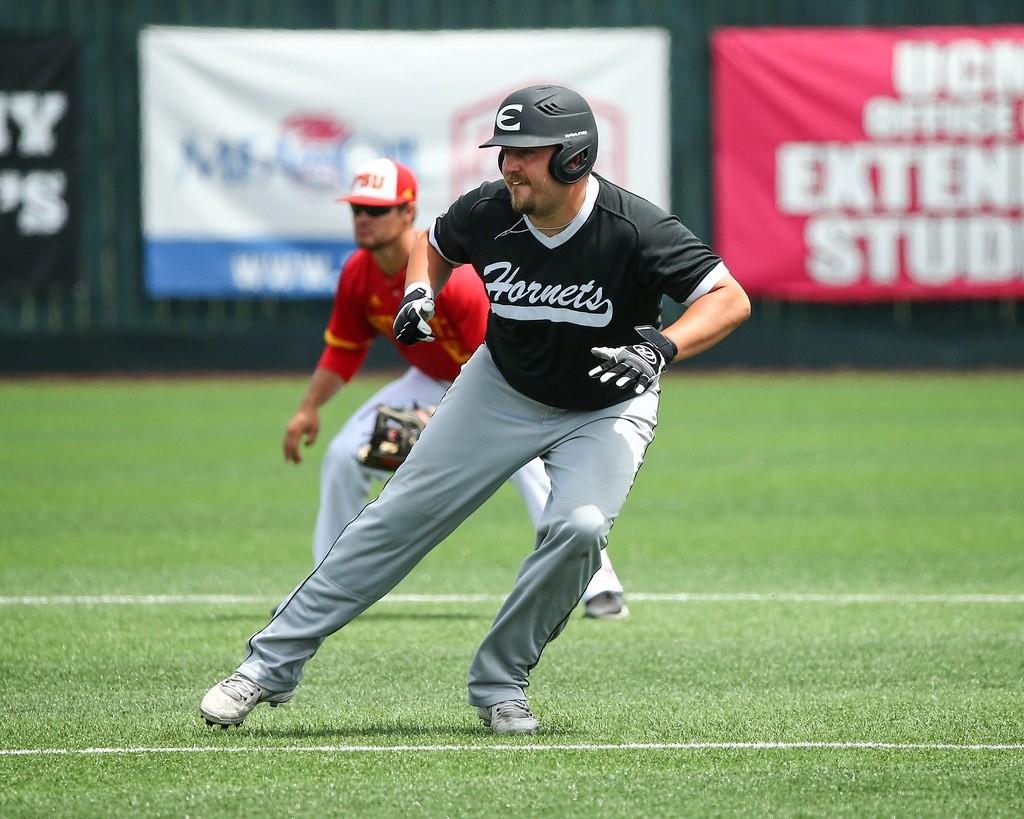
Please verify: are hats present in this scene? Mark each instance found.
[335,158,417,206]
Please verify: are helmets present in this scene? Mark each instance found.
[479,85,598,183]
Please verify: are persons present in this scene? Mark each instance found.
[196,80,750,732]
[283,157,638,623]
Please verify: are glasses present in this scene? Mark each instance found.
[351,205,391,216]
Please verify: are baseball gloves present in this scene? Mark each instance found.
[352,398,436,473]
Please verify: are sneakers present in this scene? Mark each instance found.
[585,592,629,619]
[477,700,540,735]
[200,672,294,728]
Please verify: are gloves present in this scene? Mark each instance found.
[589,325,677,394]
[393,282,436,346]
